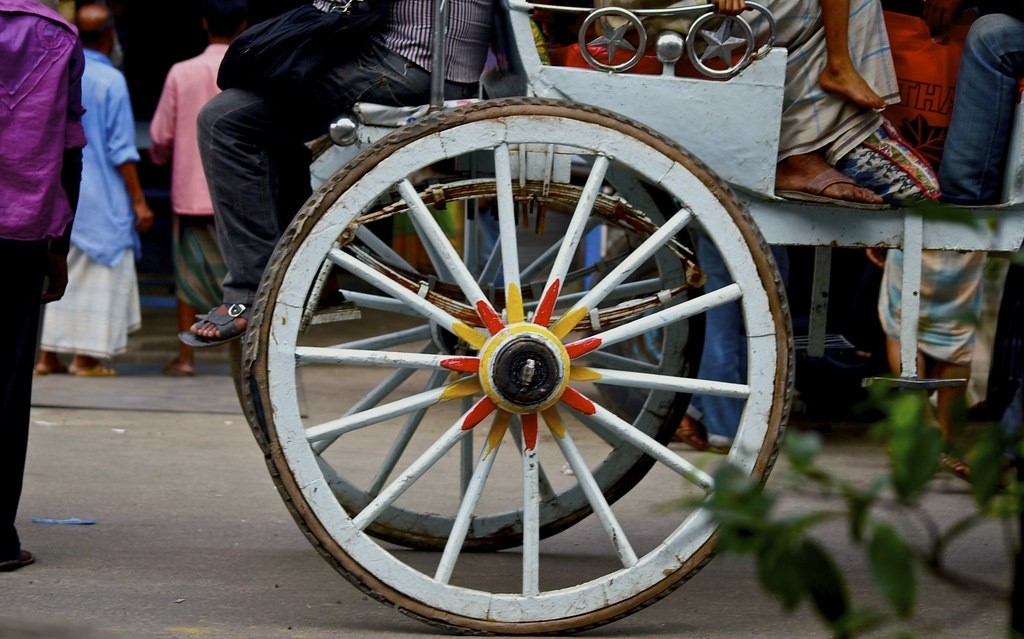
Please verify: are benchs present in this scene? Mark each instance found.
[508,0,789,207]
[311,33,519,191]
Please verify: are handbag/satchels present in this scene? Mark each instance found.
[216,3,362,96]
[831,115,941,204]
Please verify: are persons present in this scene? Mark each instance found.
[674,225,791,456]
[147,0,248,375]
[593,0,902,212]
[0,0,92,573]
[923,0,1024,207]
[865,247,1024,493]
[33,4,155,379]
[178,1,492,348]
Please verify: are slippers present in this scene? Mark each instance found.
[33,363,68,376]
[676,428,711,450]
[76,362,119,377]
[710,445,731,455]
[162,356,193,376]
[773,167,890,209]
[0,550,35,571]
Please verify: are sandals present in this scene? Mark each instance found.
[940,453,973,482]
[177,301,250,346]
